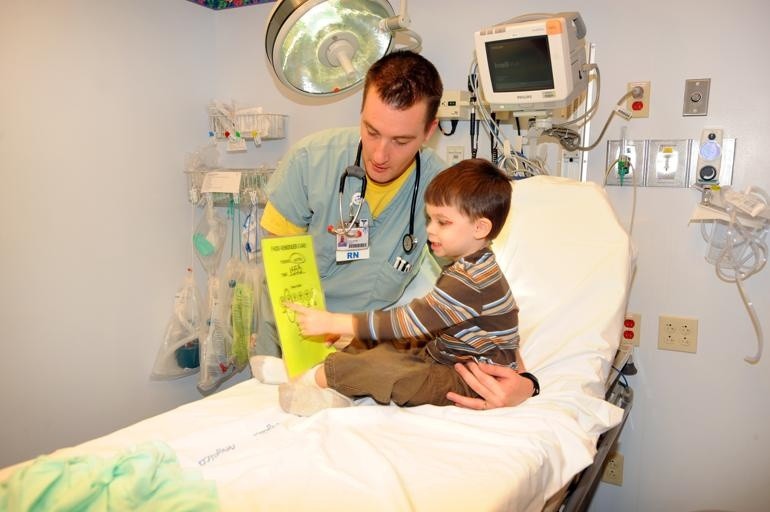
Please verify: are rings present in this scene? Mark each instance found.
[484,401,488,410]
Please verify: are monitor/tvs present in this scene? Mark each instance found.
[474,11,589,111]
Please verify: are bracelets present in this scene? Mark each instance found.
[518,371,540,397]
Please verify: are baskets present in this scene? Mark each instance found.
[208,112,288,141]
[183,165,275,209]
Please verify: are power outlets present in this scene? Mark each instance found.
[657,316,699,354]
[626,81,651,119]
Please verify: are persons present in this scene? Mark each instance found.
[256,48,541,413]
[245,156,522,420]
[338,235,347,247]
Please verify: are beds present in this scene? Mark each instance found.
[1,176,638,511]
